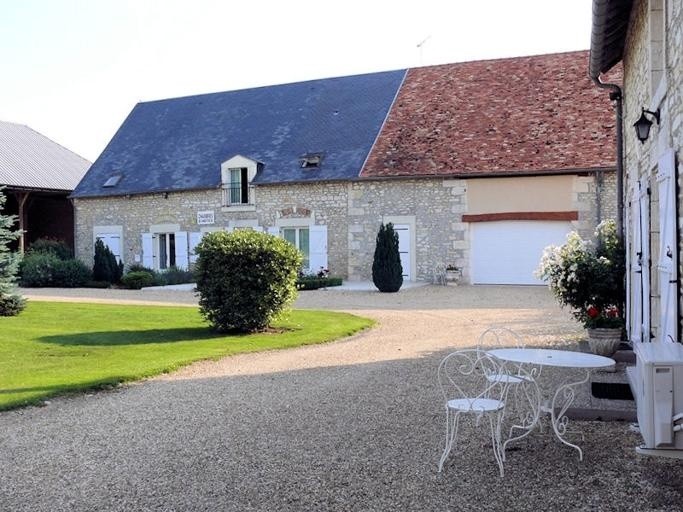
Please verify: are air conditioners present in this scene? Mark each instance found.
[634,340,682,458]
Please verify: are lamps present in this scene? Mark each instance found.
[631,107,661,146]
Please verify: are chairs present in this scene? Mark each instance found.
[436,327,543,479]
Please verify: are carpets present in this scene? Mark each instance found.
[590,381,636,401]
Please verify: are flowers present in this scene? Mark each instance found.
[532,218,629,325]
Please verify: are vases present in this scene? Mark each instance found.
[587,327,622,358]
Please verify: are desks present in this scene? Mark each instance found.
[487,348,617,462]
